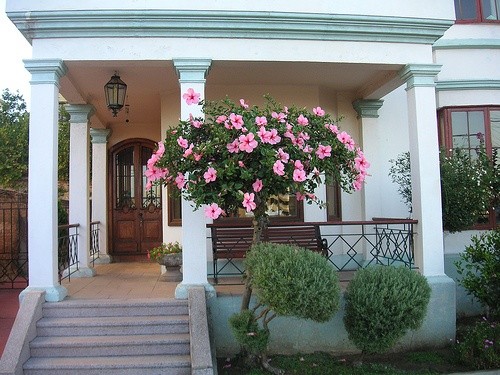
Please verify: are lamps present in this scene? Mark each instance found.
[104,70,127,118]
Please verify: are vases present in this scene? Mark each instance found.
[157,252,183,282]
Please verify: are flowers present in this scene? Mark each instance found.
[147,241,182,260]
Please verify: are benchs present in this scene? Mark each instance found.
[213,226,328,284]
